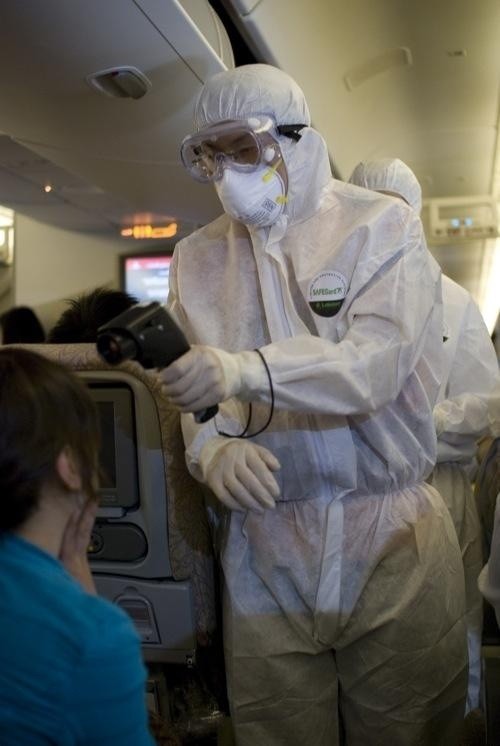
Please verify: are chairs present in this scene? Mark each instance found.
[0,341,225,671]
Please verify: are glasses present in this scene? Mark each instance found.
[180,115,299,184]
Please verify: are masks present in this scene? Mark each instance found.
[213,157,286,228]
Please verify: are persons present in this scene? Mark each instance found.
[346,158,500,746]
[47,282,142,344]
[157,60,471,746]
[0,346,158,745]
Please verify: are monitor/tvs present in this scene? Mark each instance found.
[86,390,139,507]
[119,251,174,307]
